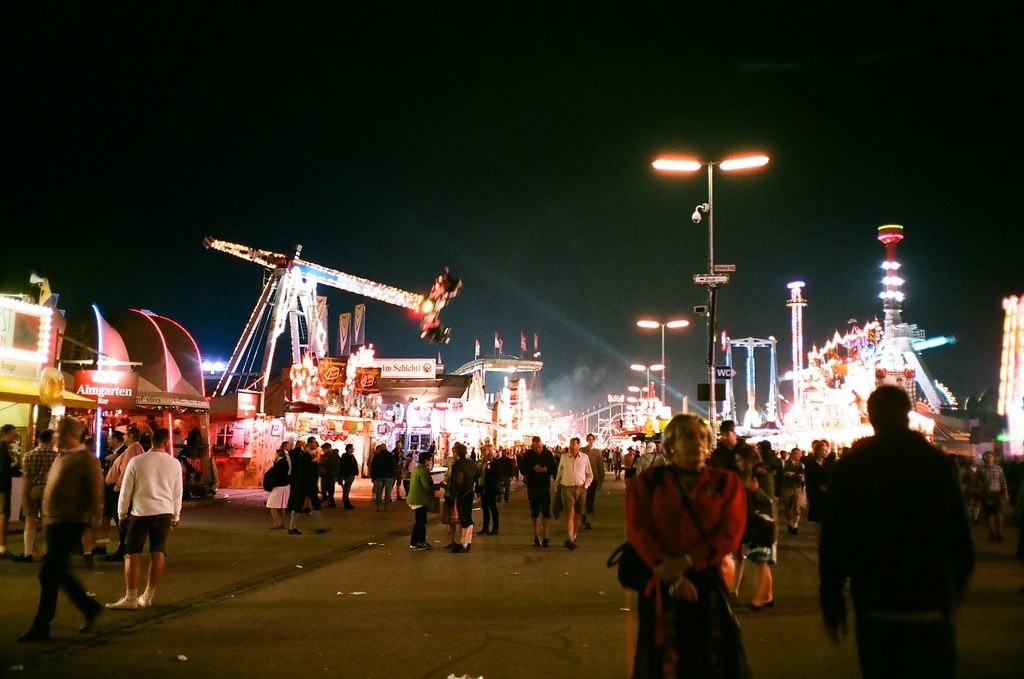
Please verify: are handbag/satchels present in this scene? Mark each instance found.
[716,555,736,595]
[607,467,662,590]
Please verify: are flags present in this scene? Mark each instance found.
[500,339,503,354]
[475,339,480,356]
[495,331,500,348]
[438,352,442,363]
[534,332,538,350]
[520,332,527,351]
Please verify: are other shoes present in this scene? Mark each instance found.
[0,544,126,562]
[745,602,773,612]
[17,622,50,643]
[270,494,408,535]
[534,536,549,548]
[565,537,577,551]
[78,603,105,632]
[478,528,498,536]
[443,540,472,553]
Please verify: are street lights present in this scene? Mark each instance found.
[636,319,691,446]
[630,363,665,420]
[626,386,649,407]
[653,153,771,453]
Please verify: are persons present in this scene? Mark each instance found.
[407,437,514,552]
[516,433,605,551]
[607,413,811,679]
[804,382,1024,679]
[0,415,204,641]
[263,437,406,534]
[217,426,233,447]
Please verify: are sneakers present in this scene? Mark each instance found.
[410,541,433,551]
[136,595,152,607]
[105,597,138,610]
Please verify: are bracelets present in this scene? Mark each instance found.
[669,575,685,597]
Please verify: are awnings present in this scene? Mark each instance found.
[0,375,97,410]
